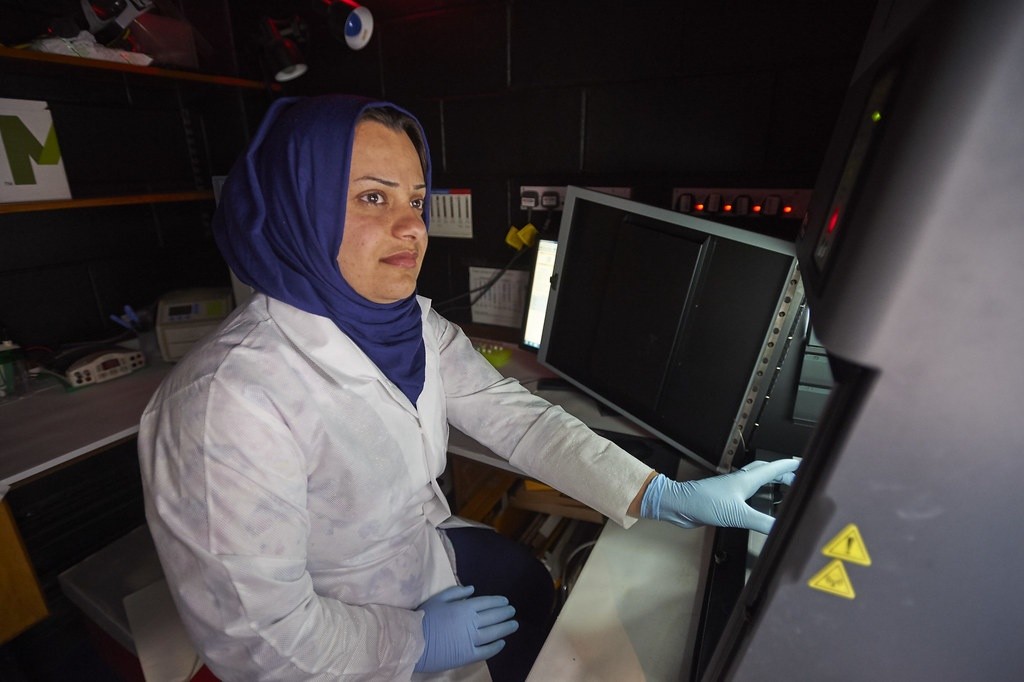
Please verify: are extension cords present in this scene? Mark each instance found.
[670,187,814,221]
[520,185,631,211]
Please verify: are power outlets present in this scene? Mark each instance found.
[673,188,812,219]
[519,186,631,212]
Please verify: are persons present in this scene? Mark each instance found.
[135,88,805,681]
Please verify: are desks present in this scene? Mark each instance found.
[0,363,175,647]
[523,516,718,682]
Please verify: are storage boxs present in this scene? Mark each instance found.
[59,522,220,682]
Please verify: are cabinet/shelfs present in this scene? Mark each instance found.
[0,0,283,212]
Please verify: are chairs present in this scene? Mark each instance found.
[124,577,221,682]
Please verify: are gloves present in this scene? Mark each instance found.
[640,455,803,535]
[412,586,519,673]
